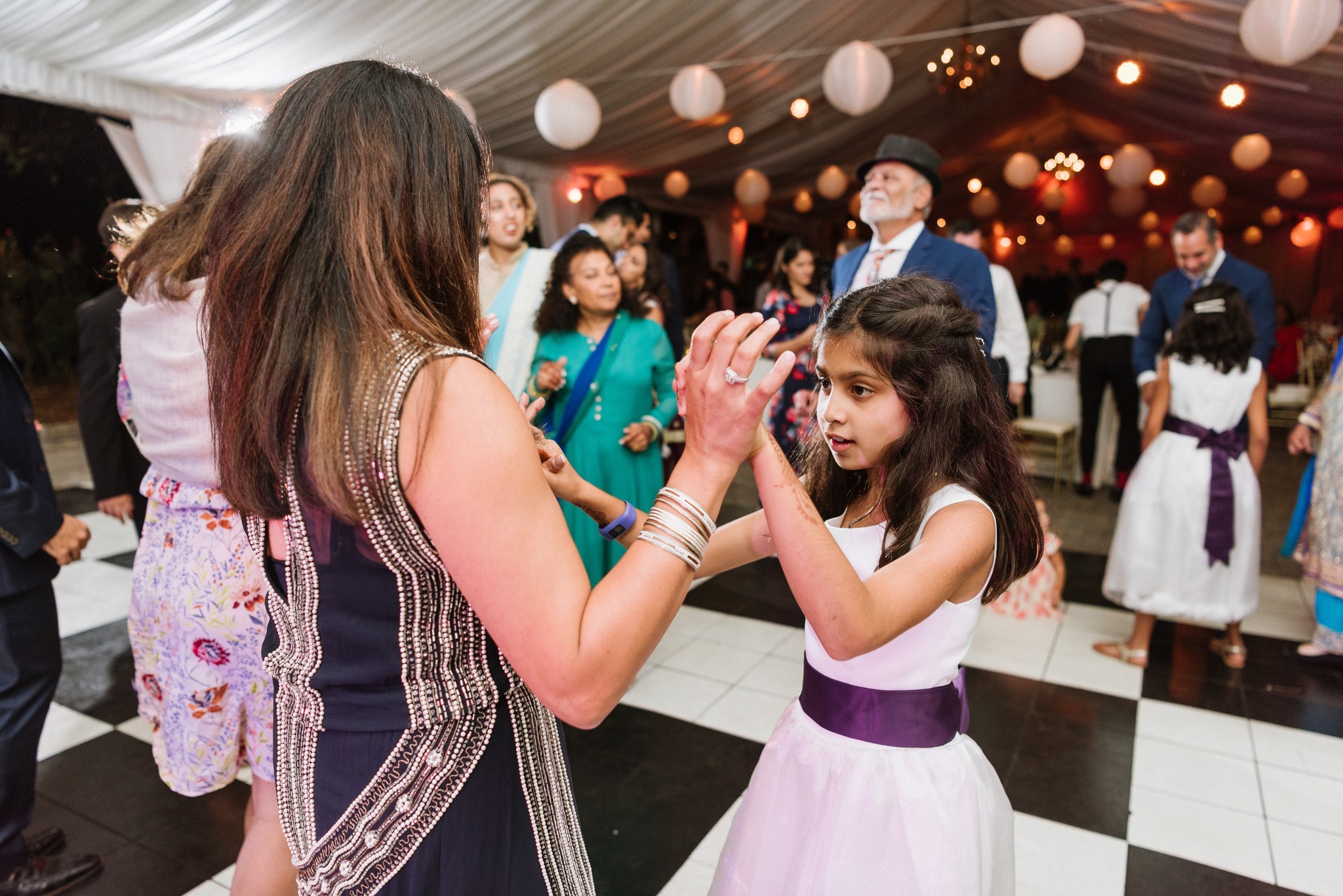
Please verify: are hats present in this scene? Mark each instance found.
[856,132,945,200]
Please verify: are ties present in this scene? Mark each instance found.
[1191,274,1206,289]
[864,247,897,288]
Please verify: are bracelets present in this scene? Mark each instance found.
[598,498,635,542]
[638,485,717,574]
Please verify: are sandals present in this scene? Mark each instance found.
[1207,636,1247,671]
[1091,635,1149,668]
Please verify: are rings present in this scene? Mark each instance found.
[725,366,748,385]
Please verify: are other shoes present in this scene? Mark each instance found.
[1107,483,1124,503]
[1071,480,1094,499]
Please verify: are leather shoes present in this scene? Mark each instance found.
[0,823,106,896]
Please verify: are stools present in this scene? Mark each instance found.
[1010,416,1079,499]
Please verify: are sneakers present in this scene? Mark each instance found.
[1295,639,1343,658]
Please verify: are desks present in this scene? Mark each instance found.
[1030,357,1148,491]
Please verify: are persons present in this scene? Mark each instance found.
[527,273,1044,896]
[471,175,1342,594]
[1093,285,1268,669]
[118,61,797,896]
[116,133,301,896]
[72,198,160,541]
[0,340,108,895]
[832,133,996,348]
[1133,210,1274,439]
[1280,338,1342,665]
[989,498,1063,617]
